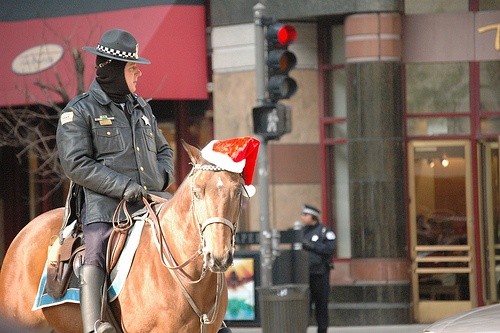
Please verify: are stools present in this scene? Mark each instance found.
[429,285,460,301]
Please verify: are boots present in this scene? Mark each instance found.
[79,265,116,333]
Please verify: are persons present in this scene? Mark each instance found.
[455,221,470,301]
[57,29,174,333]
[301,204,337,333]
[439,221,457,300]
[416,214,438,301]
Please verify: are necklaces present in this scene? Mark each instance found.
[112,101,126,111]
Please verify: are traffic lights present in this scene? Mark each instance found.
[254,104,286,138]
[266,22,299,101]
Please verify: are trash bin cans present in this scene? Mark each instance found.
[254,284,311,333]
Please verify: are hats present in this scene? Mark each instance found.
[301,204,321,220]
[81,29,151,65]
[201,136,260,198]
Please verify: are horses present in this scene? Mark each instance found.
[1,139,249,332]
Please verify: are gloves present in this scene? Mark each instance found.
[301,238,311,247]
[123,180,147,204]
